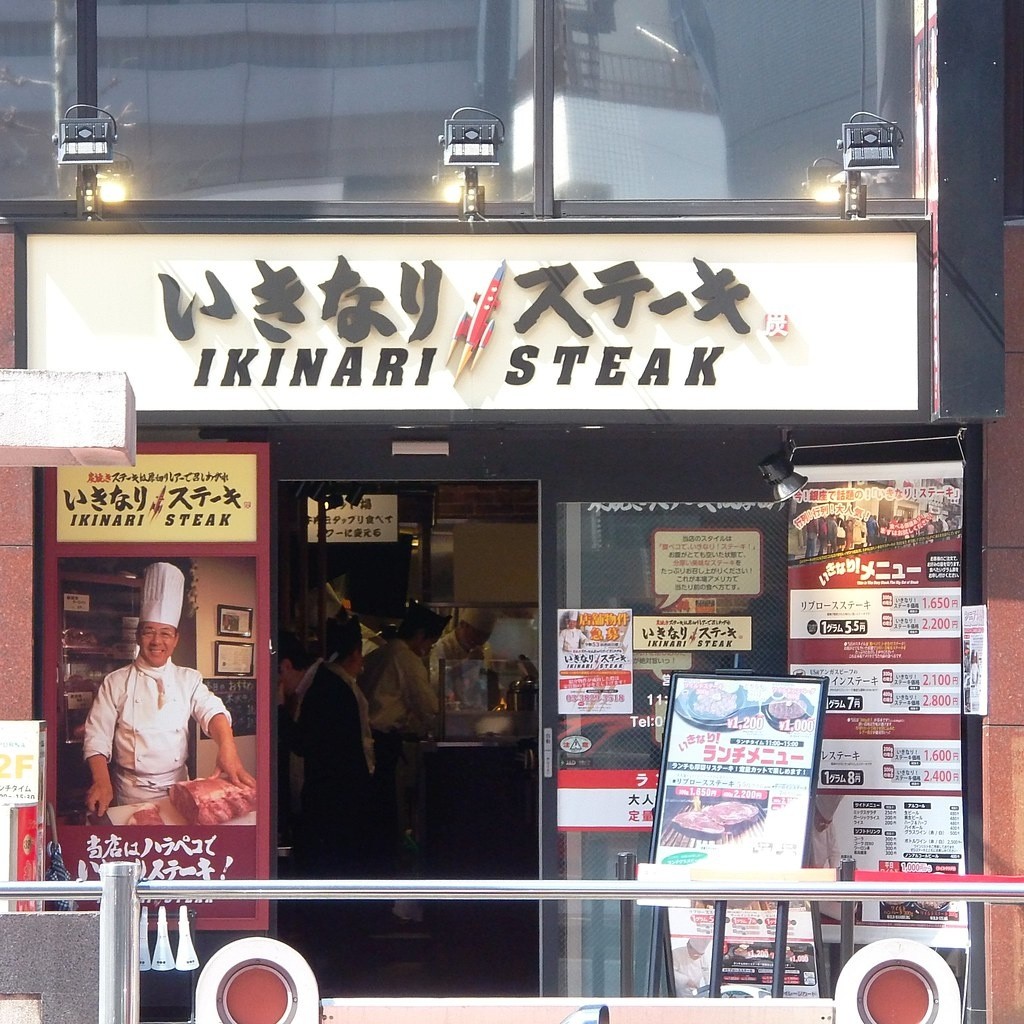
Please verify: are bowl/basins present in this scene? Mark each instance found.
[761,691,814,732]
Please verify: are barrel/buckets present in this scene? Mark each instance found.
[506,677,540,715]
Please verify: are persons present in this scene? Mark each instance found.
[272,604,452,861]
[81,562,257,818]
[559,610,588,652]
[429,604,497,701]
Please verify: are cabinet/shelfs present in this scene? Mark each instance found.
[50,559,195,821]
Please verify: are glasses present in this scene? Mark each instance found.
[135,625,176,641]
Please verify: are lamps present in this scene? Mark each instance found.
[439,118,497,222]
[758,451,808,503]
[52,120,113,222]
[392,440,450,456]
[807,156,893,203]
[835,124,899,220]
[432,172,479,204]
[100,151,135,205]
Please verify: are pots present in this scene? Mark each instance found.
[673,684,760,727]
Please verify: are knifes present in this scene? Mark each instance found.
[87,803,114,825]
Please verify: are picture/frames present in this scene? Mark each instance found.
[214,641,254,676]
[217,604,253,638]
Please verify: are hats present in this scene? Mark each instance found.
[406,598,453,635]
[137,561,187,628]
[461,605,496,635]
